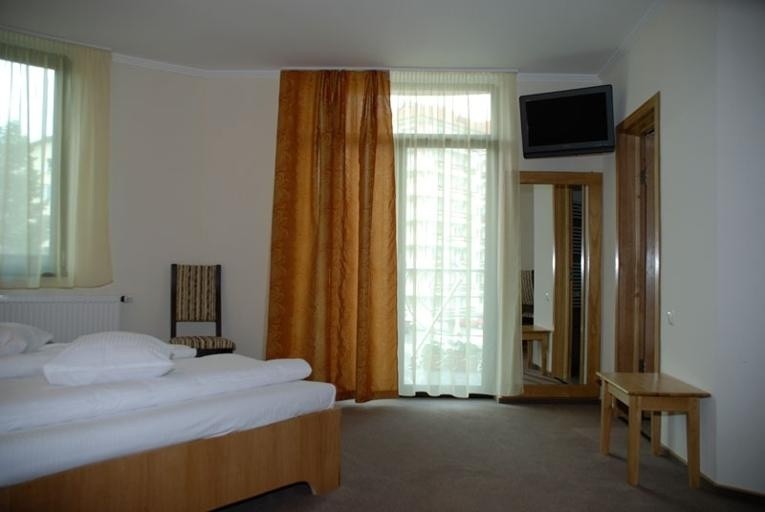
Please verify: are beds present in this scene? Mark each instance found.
[0,345,345,511]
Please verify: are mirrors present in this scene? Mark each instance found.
[520,172,600,401]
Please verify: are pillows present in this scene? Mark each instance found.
[0,322,199,386]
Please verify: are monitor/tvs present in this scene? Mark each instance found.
[519,84,615,159]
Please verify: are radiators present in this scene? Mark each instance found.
[1,294,131,344]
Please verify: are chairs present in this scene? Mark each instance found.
[167,265,235,354]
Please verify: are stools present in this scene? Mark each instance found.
[520,326,552,376]
[597,371,711,489]
